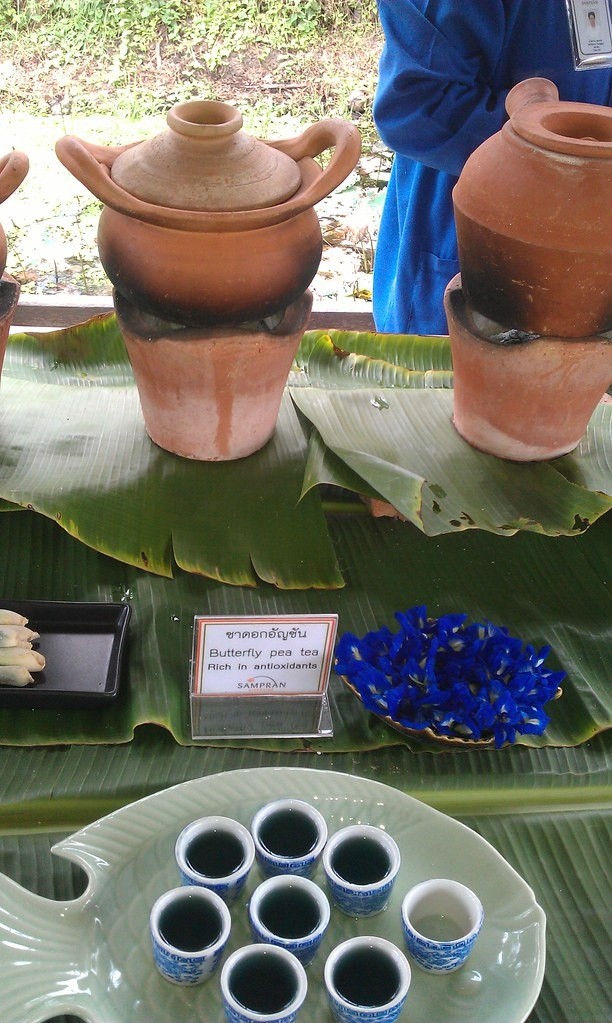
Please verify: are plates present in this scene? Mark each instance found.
[0,598,134,710]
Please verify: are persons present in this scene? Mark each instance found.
[373,0,612,336]
[586,12,602,32]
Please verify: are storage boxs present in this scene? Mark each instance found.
[0,598,132,710]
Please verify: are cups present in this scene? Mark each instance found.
[402,878,486,976]
[148,885,234,986]
[220,943,309,1023]
[248,874,330,967]
[321,824,402,918]
[323,935,412,1023]
[174,815,255,907]
[250,798,329,880]
